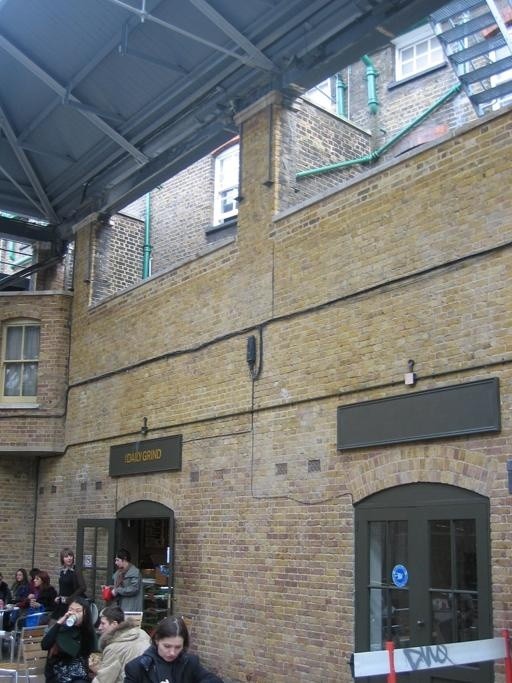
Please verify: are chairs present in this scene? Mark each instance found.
[0,613,62,682]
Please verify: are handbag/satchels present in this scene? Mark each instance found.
[18,599,37,608]
[87,651,103,672]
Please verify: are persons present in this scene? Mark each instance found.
[102,550,144,628]
[123,617,223,683]
[41,597,98,683]
[88,607,151,683]
[51,547,87,619]
[0,567,57,631]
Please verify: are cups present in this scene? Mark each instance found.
[102,587,112,601]
[66,614,77,627]
[30,599,36,609]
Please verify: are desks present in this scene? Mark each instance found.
[1,603,32,630]
